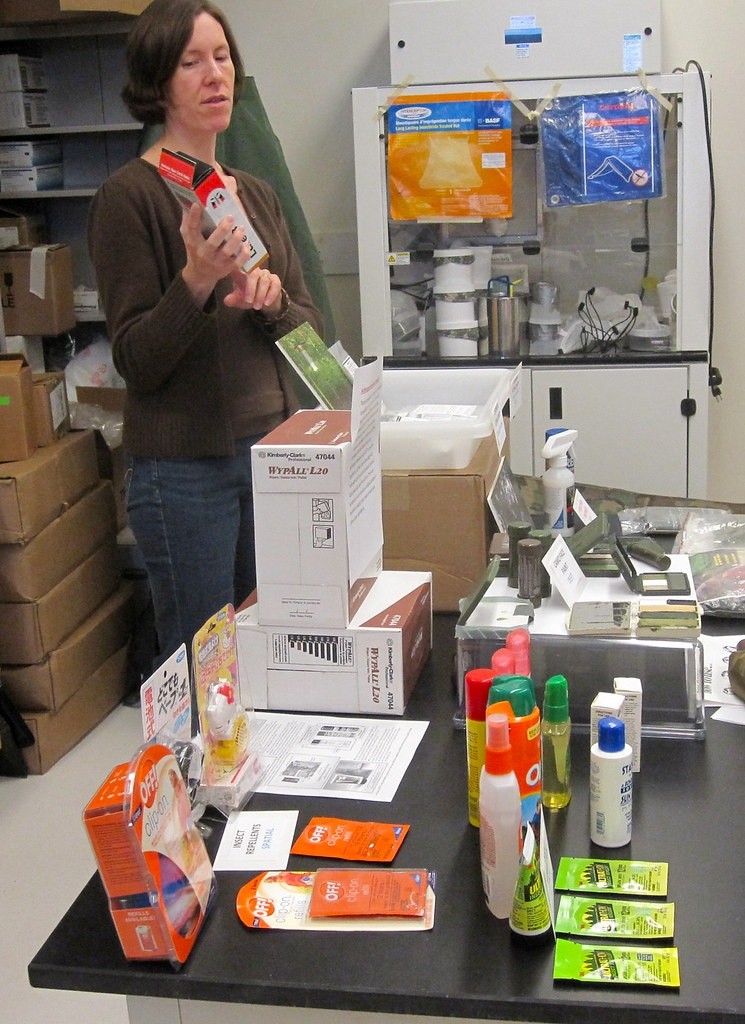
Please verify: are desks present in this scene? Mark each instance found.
[25,476,745,1024]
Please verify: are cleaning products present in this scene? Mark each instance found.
[540,428,577,538]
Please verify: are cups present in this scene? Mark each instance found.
[489,292,529,356]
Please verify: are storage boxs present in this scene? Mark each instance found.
[313,368,514,470]
[380,416,510,613]
[248,408,384,631]
[451,554,708,742]
[613,677,642,773]
[79,742,221,972]
[608,532,691,596]
[0,0,153,778]
[564,512,621,578]
[589,692,626,746]
[233,571,435,716]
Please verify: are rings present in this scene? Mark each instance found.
[263,275,272,281]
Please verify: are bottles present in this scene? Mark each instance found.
[590,718,632,847]
[465,629,572,919]
[509,522,531,588]
[528,530,552,597]
[516,539,541,608]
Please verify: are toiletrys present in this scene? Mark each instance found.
[590,717,632,849]
[465,628,572,954]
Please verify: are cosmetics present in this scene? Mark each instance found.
[590,676,642,773]
[509,821,551,935]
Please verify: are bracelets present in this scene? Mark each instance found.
[258,288,290,322]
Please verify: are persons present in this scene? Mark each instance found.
[86,0,326,681]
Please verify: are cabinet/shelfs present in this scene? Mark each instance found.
[0,15,136,571]
